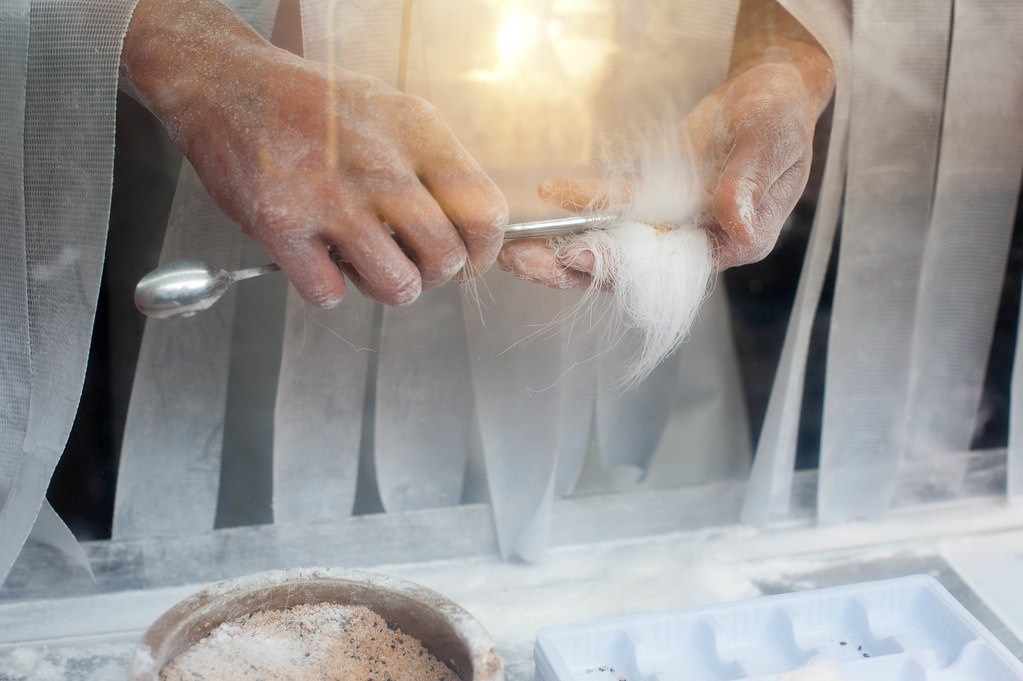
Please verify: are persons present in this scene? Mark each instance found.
[26,0,835,529]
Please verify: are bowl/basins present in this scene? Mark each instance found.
[127,565,507,680]
